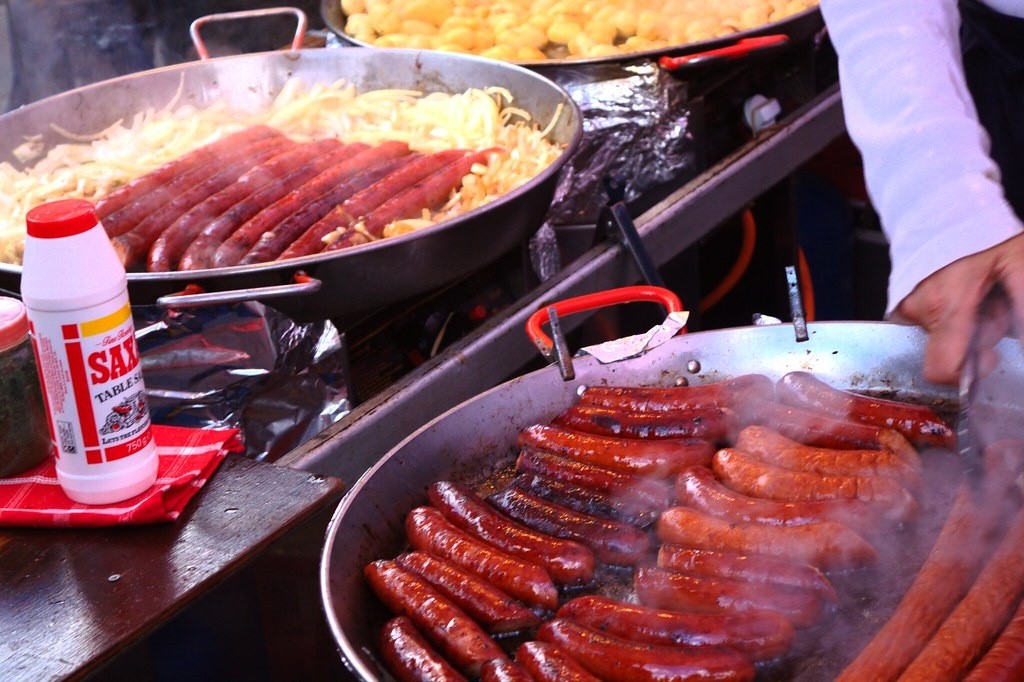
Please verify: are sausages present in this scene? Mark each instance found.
[93,125,502,275]
[366,373,1024,682]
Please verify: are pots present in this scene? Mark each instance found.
[0,7,584,324]
[316,284,1024,682]
[320,0,821,74]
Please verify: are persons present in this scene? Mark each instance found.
[817,0,1024,387]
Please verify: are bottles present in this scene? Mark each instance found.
[20,199,159,504]
